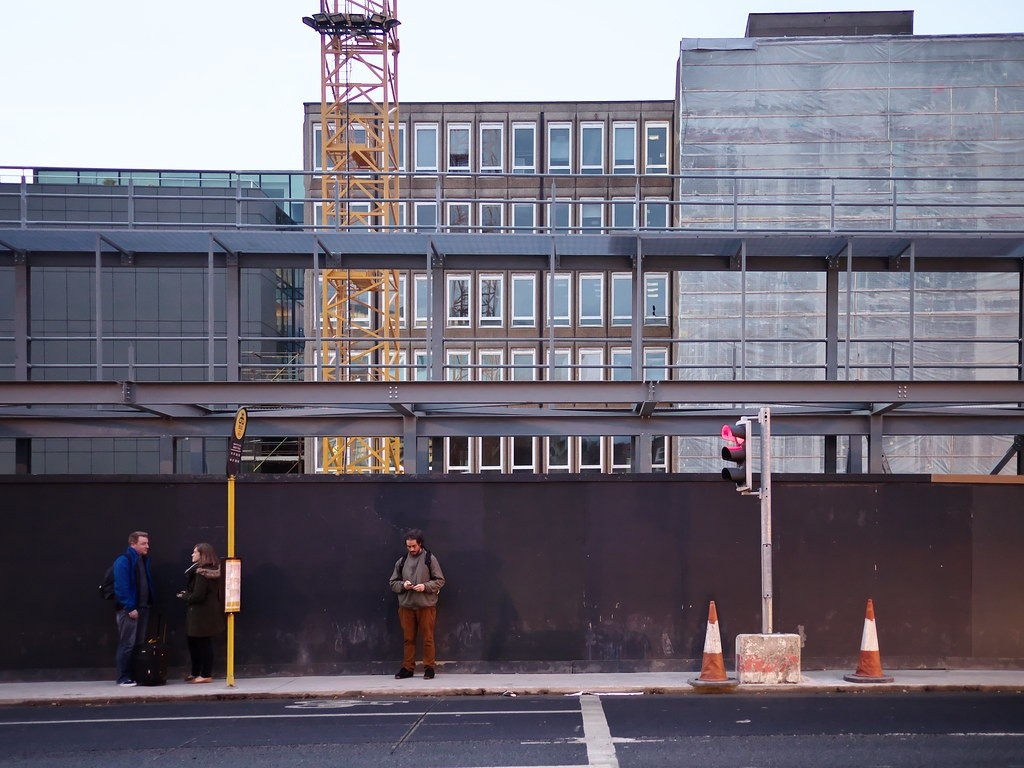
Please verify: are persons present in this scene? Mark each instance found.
[177,543,225,683]
[389,529,445,678]
[114,531,150,686]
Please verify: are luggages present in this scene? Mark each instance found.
[137,610,168,686]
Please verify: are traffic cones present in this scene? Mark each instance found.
[842,596,896,685]
[685,599,740,686]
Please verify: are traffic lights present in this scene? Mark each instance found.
[719,420,755,492]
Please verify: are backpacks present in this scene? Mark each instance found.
[98,553,132,600]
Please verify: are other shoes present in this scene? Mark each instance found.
[185,674,196,681]
[191,678,212,683]
[118,679,137,686]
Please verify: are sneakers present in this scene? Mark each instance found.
[395,667,413,679]
[424,668,434,678]
